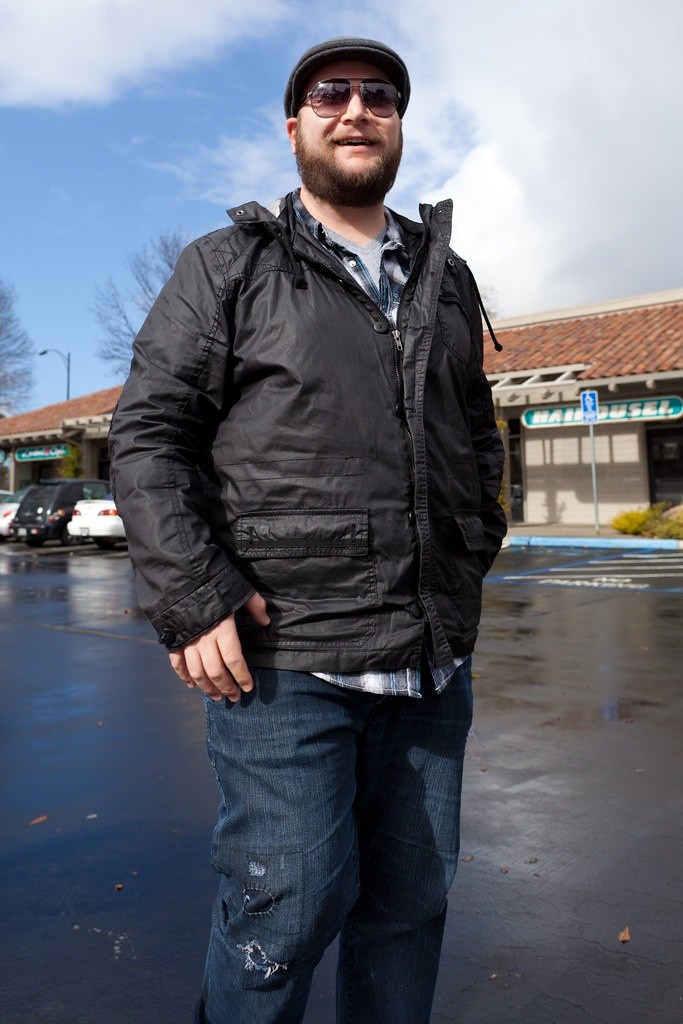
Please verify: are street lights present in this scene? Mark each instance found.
[39,349,71,399]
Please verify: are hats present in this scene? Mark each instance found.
[284,38,414,114]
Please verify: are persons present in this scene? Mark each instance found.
[103,38,511,1023]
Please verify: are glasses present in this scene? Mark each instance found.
[298,74,401,117]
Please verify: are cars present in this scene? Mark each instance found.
[66,492,127,550]
[7,478,112,549]
